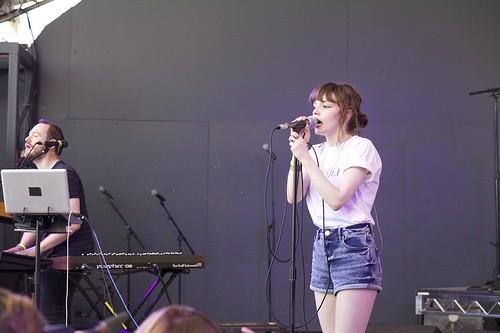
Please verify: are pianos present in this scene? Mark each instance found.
[48,252,205,272]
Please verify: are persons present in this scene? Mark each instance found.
[287,83,383,333]
[131,305,256,333]
[2,119,95,327]
[0,285,46,333]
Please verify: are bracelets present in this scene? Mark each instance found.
[289,163,298,172]
[18,243,27,250]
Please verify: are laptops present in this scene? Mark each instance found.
[1,169,82,224]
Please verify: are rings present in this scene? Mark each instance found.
[294,135,300,139]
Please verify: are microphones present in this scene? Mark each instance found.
[151,189,166,201]
[277,116,318,130]
[37,140,68,147]
[262,143,276,160]
[99,186,114,199]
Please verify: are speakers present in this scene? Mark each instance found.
[365,323,441,333]
